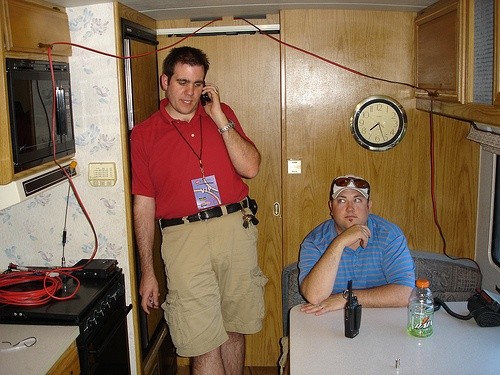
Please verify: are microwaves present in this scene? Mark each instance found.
[6,55,77,174]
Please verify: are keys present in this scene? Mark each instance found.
[242,215,259,229]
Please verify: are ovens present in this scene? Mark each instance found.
[78,298,133,375]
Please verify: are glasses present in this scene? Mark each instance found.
[330,177,370,202]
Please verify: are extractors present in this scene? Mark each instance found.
[0,160,81,212]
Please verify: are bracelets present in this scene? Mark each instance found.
[218,121,233,133]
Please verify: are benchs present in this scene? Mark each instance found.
[281,250,486,375]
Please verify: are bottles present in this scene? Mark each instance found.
[407,279,434,338]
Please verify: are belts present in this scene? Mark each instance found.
[161,199,248,228]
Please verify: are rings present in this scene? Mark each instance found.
[320,305,323,309]
[215,90,217,92]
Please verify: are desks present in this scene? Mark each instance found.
[290,302,500,375]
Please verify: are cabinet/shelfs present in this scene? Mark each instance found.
[0,324,81,375]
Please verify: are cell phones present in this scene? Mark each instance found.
[201,90,211,106]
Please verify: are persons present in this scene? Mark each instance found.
[284,176,416,375]
[131,46,269,374]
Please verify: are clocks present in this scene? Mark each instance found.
[349,95,408,152]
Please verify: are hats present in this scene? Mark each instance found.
[332,175,369,200]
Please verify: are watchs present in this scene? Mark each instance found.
[343,290,353,299]
